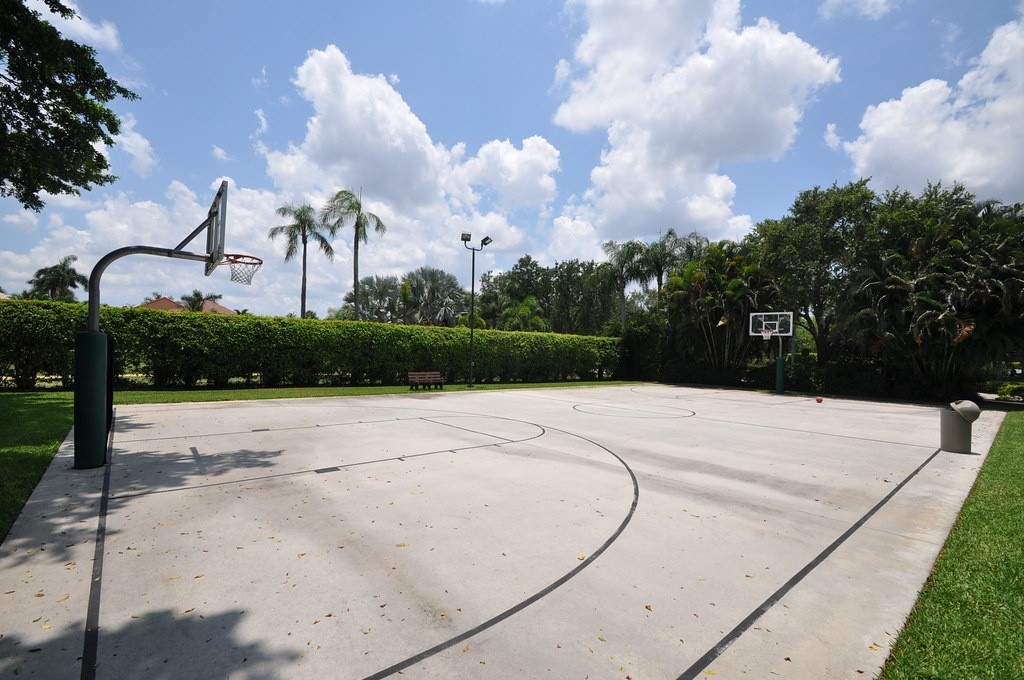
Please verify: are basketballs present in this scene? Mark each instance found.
[816,396,823,403]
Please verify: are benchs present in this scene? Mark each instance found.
[407,372,445,391]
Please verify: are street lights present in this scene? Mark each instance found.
[460,231,493,388]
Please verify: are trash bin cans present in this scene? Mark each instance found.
[940,399,982,453]
